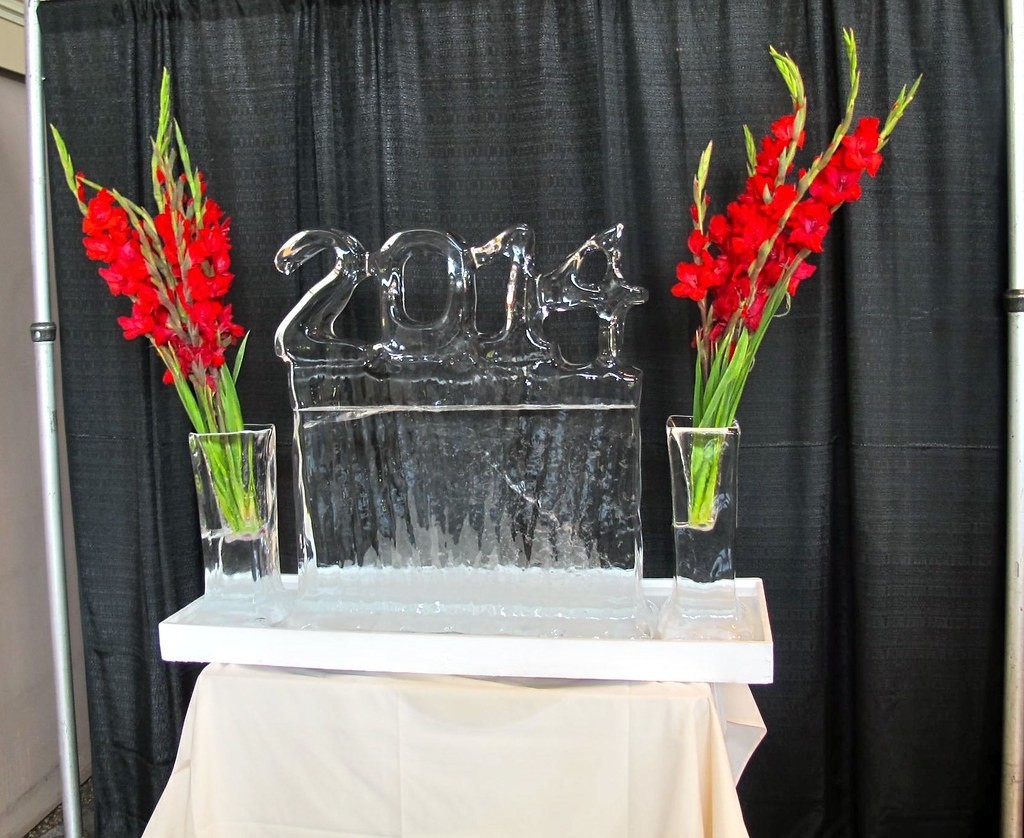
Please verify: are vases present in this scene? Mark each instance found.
[183,423,290,627]
[665,413,742,622]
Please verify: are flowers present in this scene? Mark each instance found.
[672,25,925,525]
[51,66,265,541]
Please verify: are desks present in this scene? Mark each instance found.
[157,566,778,838]
